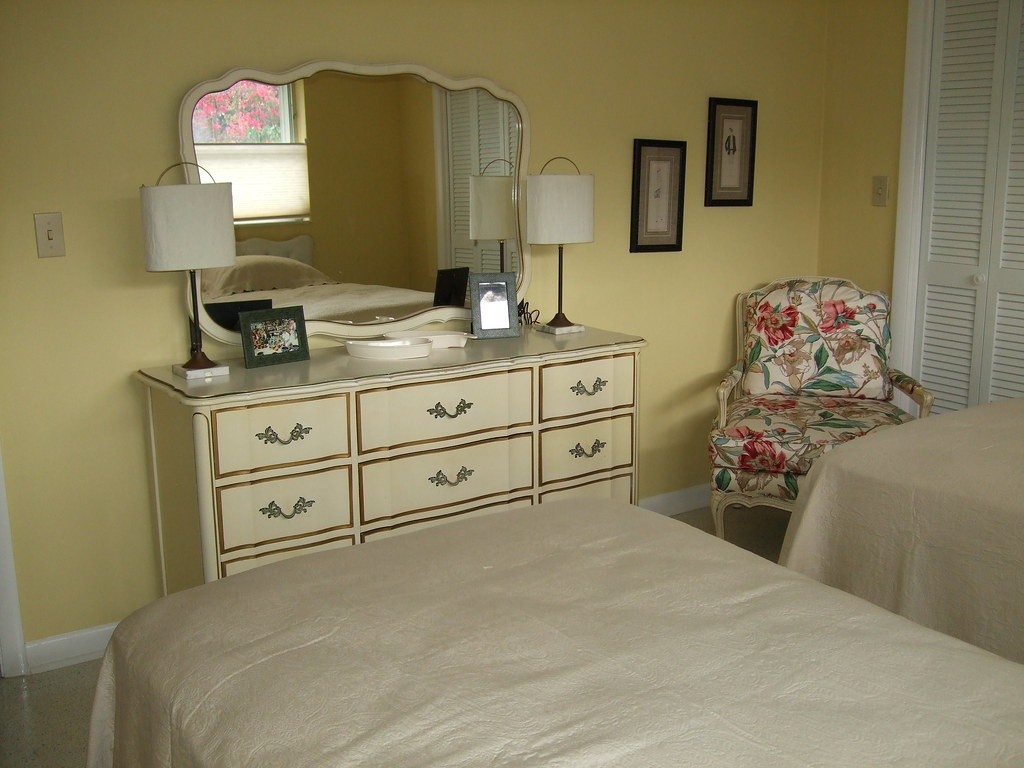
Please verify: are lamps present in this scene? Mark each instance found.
[524,157,593,334]
[468,158,517,273]
[141,162,237,381]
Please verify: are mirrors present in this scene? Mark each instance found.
[179,59,530,344]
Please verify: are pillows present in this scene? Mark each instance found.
[200,253,340,298]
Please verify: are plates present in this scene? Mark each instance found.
[383,330,477,348]
[345,338,432,358]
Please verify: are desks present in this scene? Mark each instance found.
[135,322,648,596]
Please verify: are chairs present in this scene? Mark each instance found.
[709,276,932,549]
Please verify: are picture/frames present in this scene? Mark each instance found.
[238,306,310,369]
[704,97,759,207]
[630,138,686,253]
[469,272,520,339]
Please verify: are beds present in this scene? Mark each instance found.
[87,500,1024,768]
[205,234,436,322]
[778,394,1024,662]
